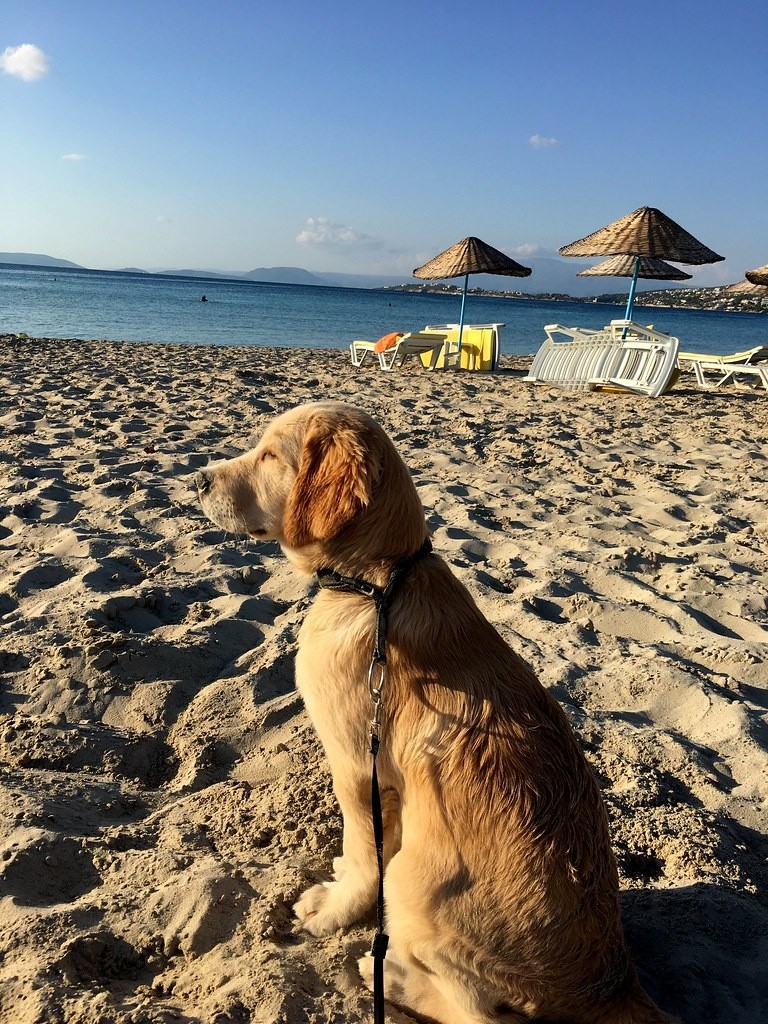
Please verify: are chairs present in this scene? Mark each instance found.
[349,332,448,371]
[524,320,680,398]
[677,346,768,390]
[419,323,507,372]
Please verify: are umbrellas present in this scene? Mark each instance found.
[412,237,532,372]
[745,264,768,286]
[560,206,726,340]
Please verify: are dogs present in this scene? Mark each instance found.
[190,397,700,1024]
